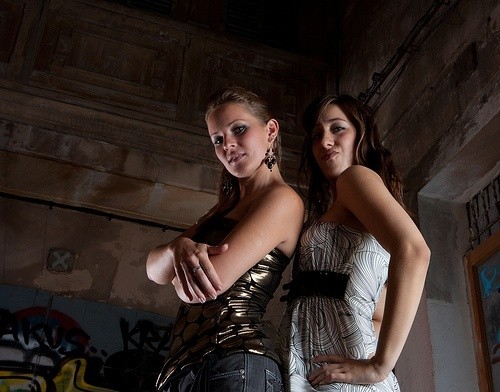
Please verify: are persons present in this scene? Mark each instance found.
[146,87,307,392]
[274,92,433,392]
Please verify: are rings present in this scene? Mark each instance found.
[193,266,202,273]
